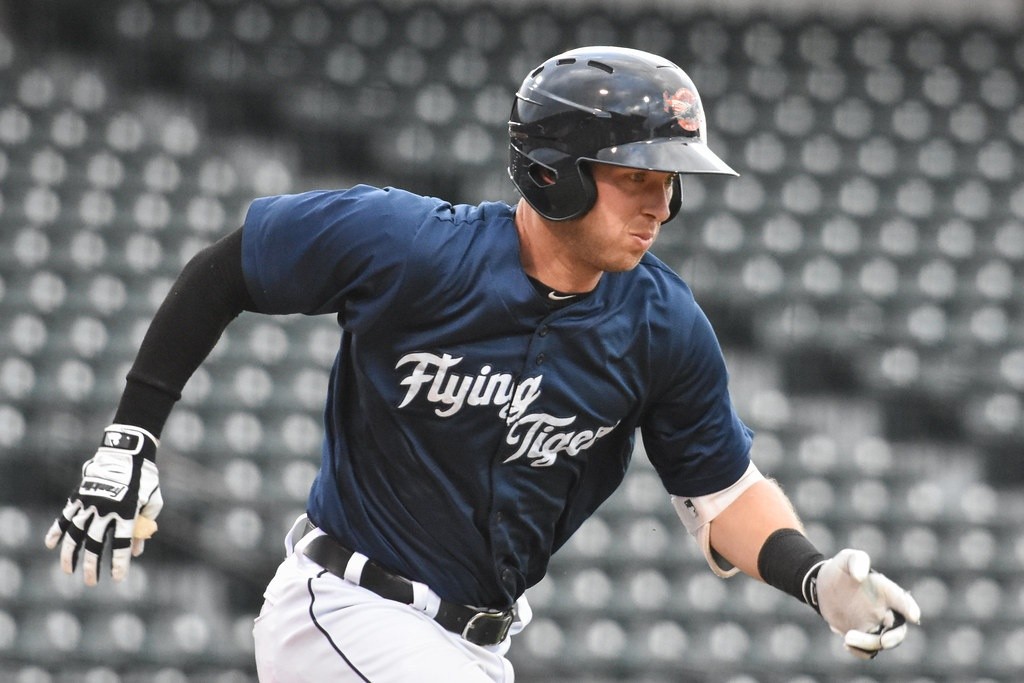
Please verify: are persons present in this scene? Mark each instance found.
[43,44,922,683]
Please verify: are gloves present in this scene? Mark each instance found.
[44,425,163,586]
[801,549,921,661]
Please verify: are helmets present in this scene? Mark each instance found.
[508,46,740,221]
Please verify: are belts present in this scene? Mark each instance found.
[295,522,516,646]
